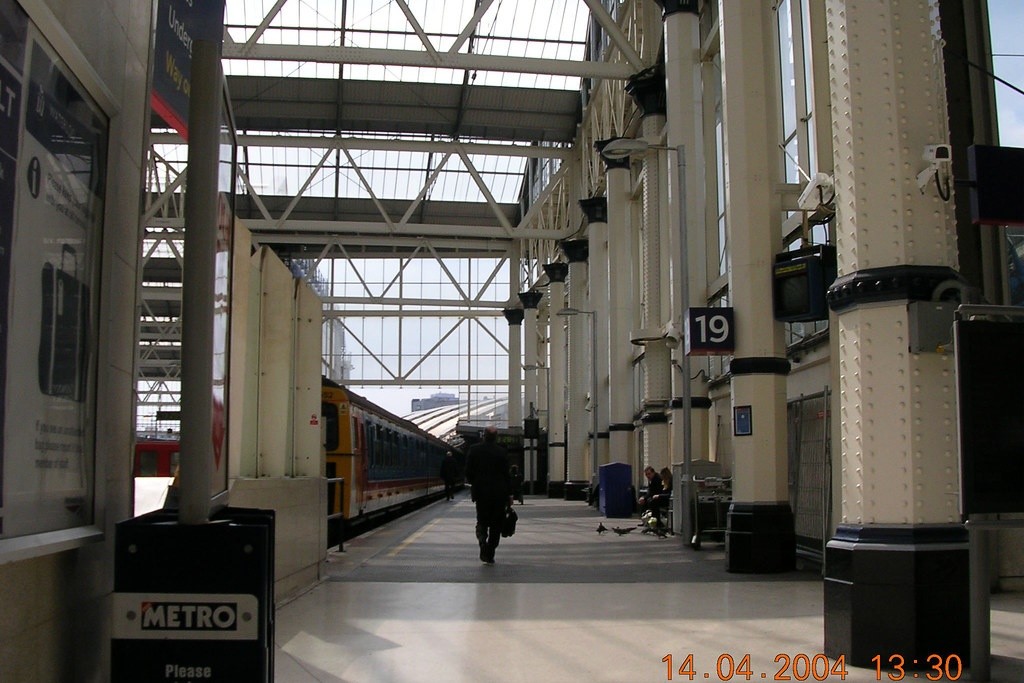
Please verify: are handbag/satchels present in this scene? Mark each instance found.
[502,506,518,538]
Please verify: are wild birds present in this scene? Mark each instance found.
[612,522,668,539]
[596,521,607,535]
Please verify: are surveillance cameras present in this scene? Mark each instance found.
[922,145,952,162]
[798,173,831,210]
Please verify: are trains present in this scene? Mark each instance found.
[321,374,472,550]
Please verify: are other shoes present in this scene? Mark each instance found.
[480,543,489,560]
[451,495,454,499]
[637,522,649,527]
[484,559,495,565]
[447,496,450,501]
[652,521,665,528]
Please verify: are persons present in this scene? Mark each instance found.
[471,427,513,563]
[646,467,673,528]
[444,451,458,502]
[637,466,662,527]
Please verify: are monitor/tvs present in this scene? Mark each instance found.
[773,255,823,320]
